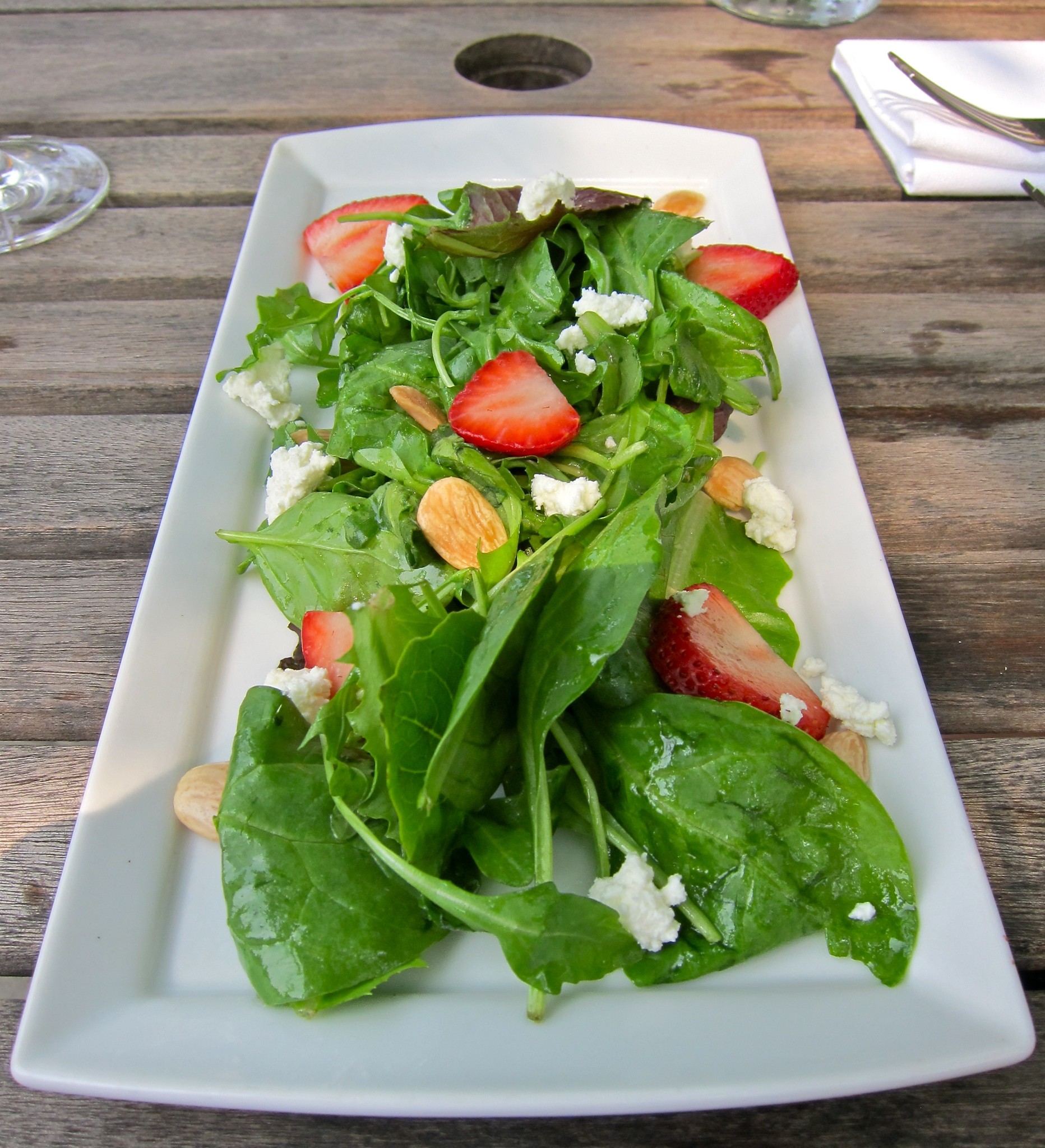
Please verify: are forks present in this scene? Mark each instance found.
[886,49,1045,145]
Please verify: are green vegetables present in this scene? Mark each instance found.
[211,171,919,1018]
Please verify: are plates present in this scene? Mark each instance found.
[11,111,1034,1122]
